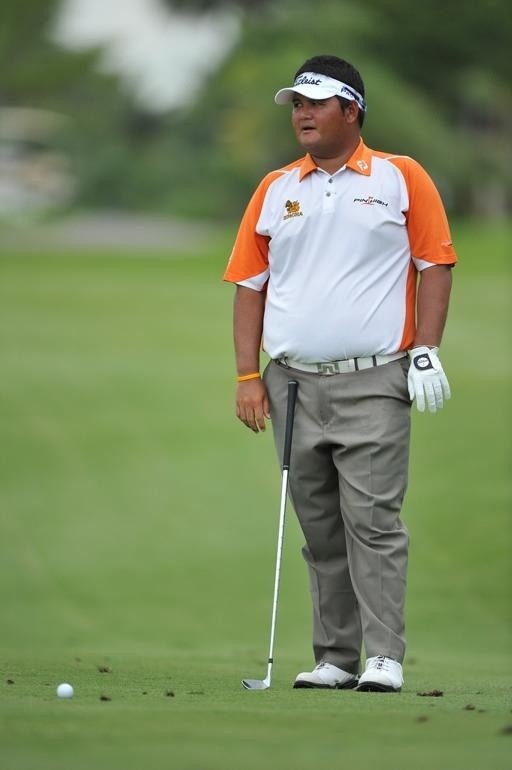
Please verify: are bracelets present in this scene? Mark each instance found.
[236,373,262,382]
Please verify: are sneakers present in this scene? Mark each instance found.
[358,655,404,693]
[294,663,360,689]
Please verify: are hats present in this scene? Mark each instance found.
[273,70,366,113]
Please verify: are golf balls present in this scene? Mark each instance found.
[57,684,74,700]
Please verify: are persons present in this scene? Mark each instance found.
[222,56,457,691]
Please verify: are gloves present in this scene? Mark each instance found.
[404,344,452,414]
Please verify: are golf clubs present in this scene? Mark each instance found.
[241,380,297,690]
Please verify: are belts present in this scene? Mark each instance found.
[275,347,408,376]
